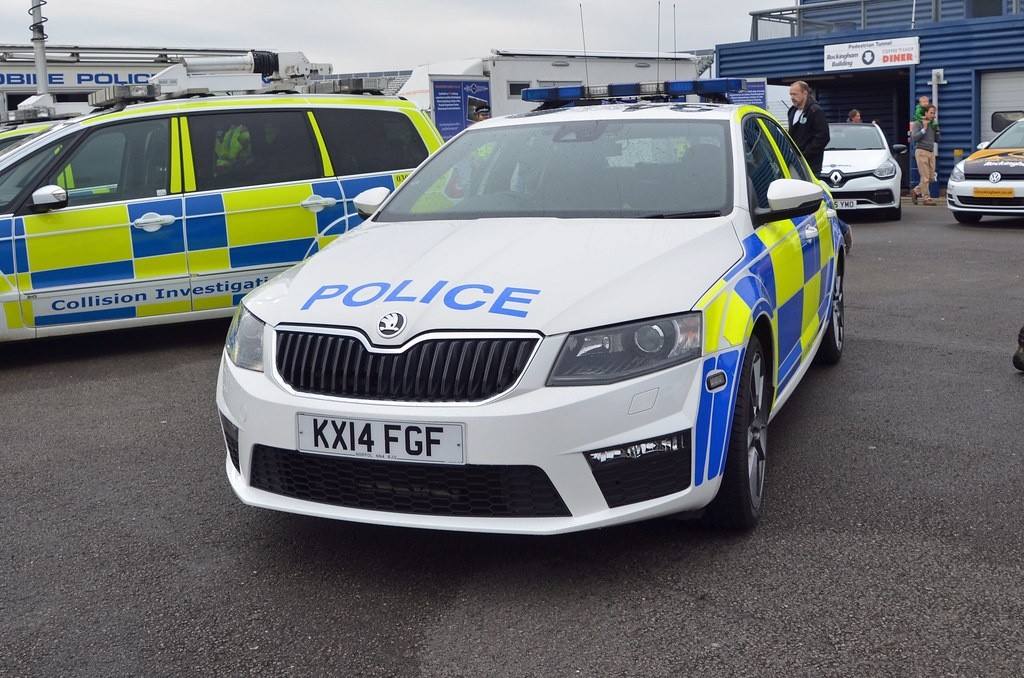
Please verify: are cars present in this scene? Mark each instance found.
[814,120,908,222]
[0,81,463,341]
[943,117,1024,224]
[216,76,850,533]
[0,94,162,204]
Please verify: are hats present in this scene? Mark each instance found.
[475,105,491,115]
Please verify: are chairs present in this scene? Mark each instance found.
[673,143,757,212]
[531,143,620,214]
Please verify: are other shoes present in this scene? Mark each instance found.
[1013,344,1024,369]
[911,190,918,205]
[923,199,937,206]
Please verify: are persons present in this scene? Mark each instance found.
[910,105,941,206]
[217,113,252,178]
[1013,327,1024,371]
[912,96,938,130]
[787,81,852,256]
[848,109,861,123]
[469,105,496,186]
